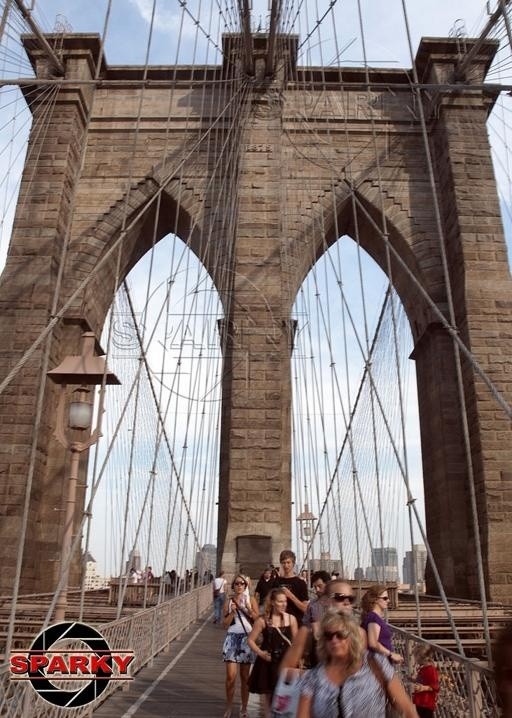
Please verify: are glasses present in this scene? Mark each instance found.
[329,593,355,604]
[323,630,352,640]
[235,581,245,585]
[377,597,388,601]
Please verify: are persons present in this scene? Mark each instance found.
[131,551,440,717]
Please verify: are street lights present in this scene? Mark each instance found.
[295,501,320,590]
[46,328,122,625]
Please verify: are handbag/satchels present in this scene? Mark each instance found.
[367,651,405,718]
[214,589,219,597]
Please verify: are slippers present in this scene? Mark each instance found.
[224,710,231,718]
[239,711,248,718]
